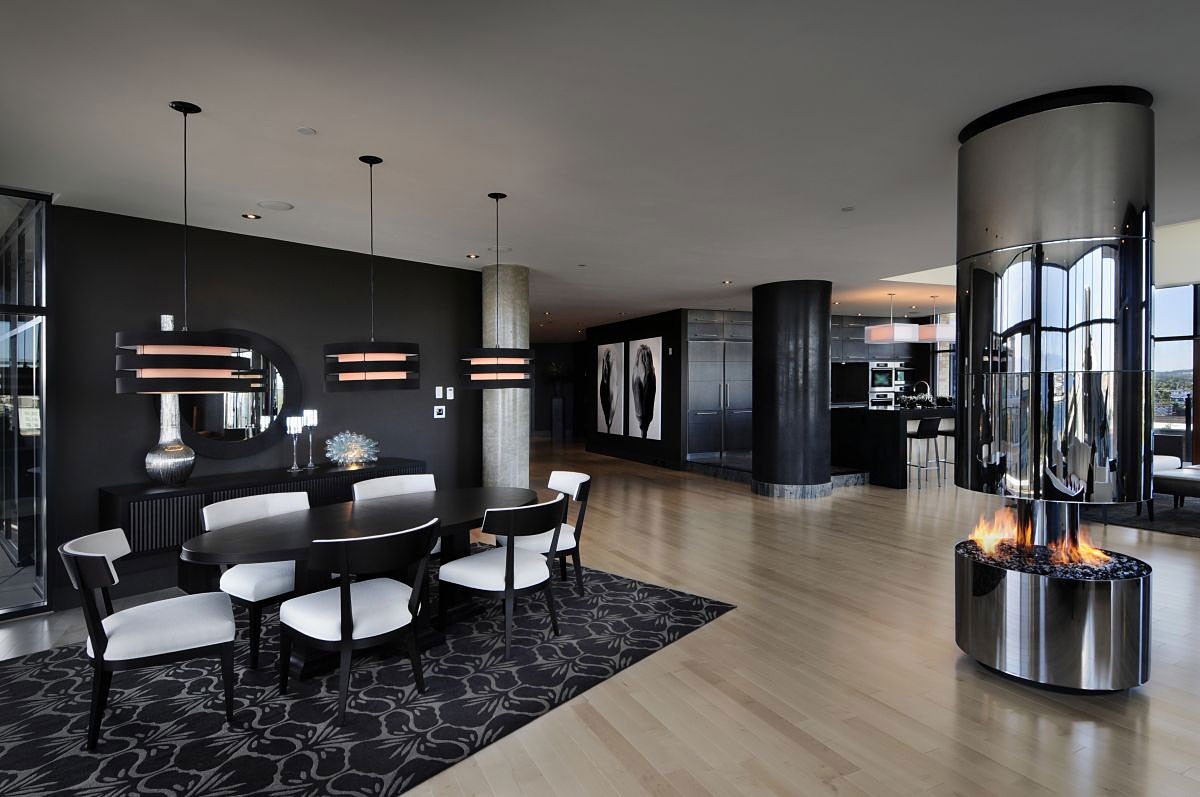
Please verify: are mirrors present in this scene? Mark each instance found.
[154,327,302,460]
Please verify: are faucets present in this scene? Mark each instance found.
[912,380,930,394]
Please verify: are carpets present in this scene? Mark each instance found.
[1079,492,1200,538]
[0,564,738,797]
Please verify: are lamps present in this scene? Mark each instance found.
[918,296,956,344]
[865,293,918,345]
[324,157,421,391]
[231,369,266,393]
[459,194,533,388]
[114,109,251,397]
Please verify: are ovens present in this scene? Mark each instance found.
[869,362,916,406]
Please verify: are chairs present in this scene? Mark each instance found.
[442,493,565,660]
[278,518,441,727]
[497,470,593,598]
[351,473,442,585]
[59,528,236,752]
[199,491,340,670]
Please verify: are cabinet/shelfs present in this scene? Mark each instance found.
[829,316,915,362]
[687,309,752,458]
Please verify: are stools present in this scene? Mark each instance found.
[1147,463,1200,522]
[925,429,955,484]
[906,417,943,490]
[1153,455,1181,508]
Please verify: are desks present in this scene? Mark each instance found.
[180,487,539,669]
[98,457,426,563]
